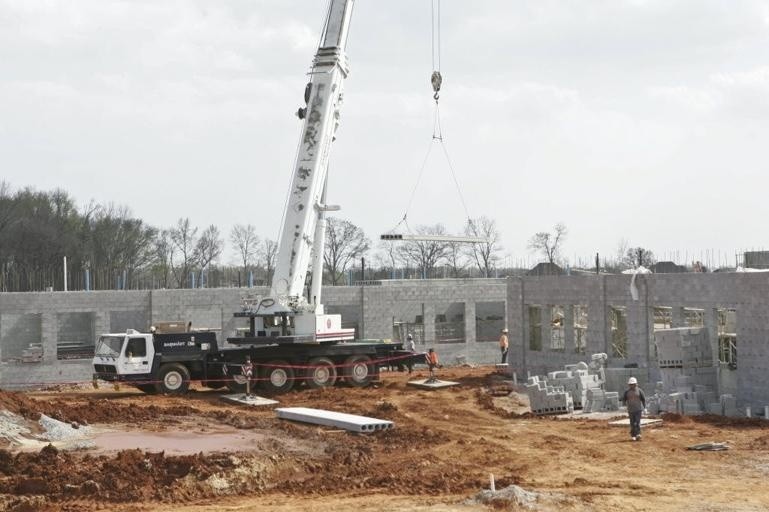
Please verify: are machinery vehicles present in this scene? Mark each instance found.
[89,1,452,399]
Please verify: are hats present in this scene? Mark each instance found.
[502,329,508,332]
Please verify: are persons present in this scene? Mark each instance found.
[618,376,647,443]
[406,333,417,376]
[425,348,438,384]
[499,327,508,364]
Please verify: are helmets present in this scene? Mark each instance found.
[628,377,637,384]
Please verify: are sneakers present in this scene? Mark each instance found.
[632,434,641,441]
